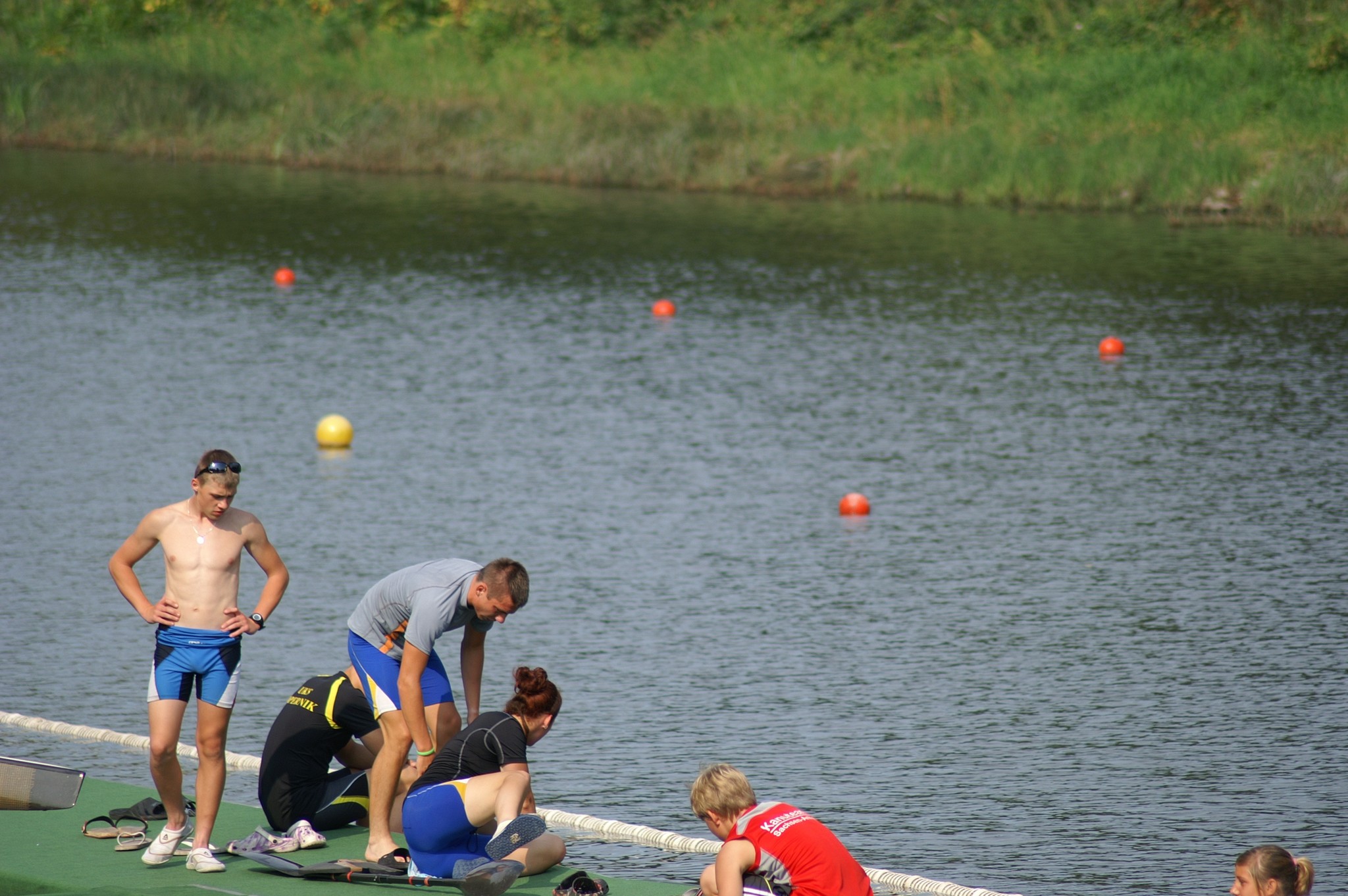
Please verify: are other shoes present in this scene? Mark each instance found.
[452,858,490,879]
[141,815,194,866]
[485,814,546,860]
[186,848,226,873]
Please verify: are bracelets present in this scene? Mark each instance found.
[416,746,435,756]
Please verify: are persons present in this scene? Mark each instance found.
[401,663,567,879]
[346,555,530,871]
[689,763,876,896]
[1229,843,1316,896]
[107,448,290,873]
[257,661,419,834]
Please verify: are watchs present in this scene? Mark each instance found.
[248,613,266,631]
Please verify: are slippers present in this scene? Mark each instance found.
[552,870,609,896]
[173,838,227,855]
[81,815,148,839]
[109,795,196,820]
[281,819,326,849]
[115,831,151,850]
[227,826,299,853]
[378,848,411,869]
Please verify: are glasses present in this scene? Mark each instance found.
[195,462,241,478]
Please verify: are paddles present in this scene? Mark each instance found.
[237,851,526,896]
